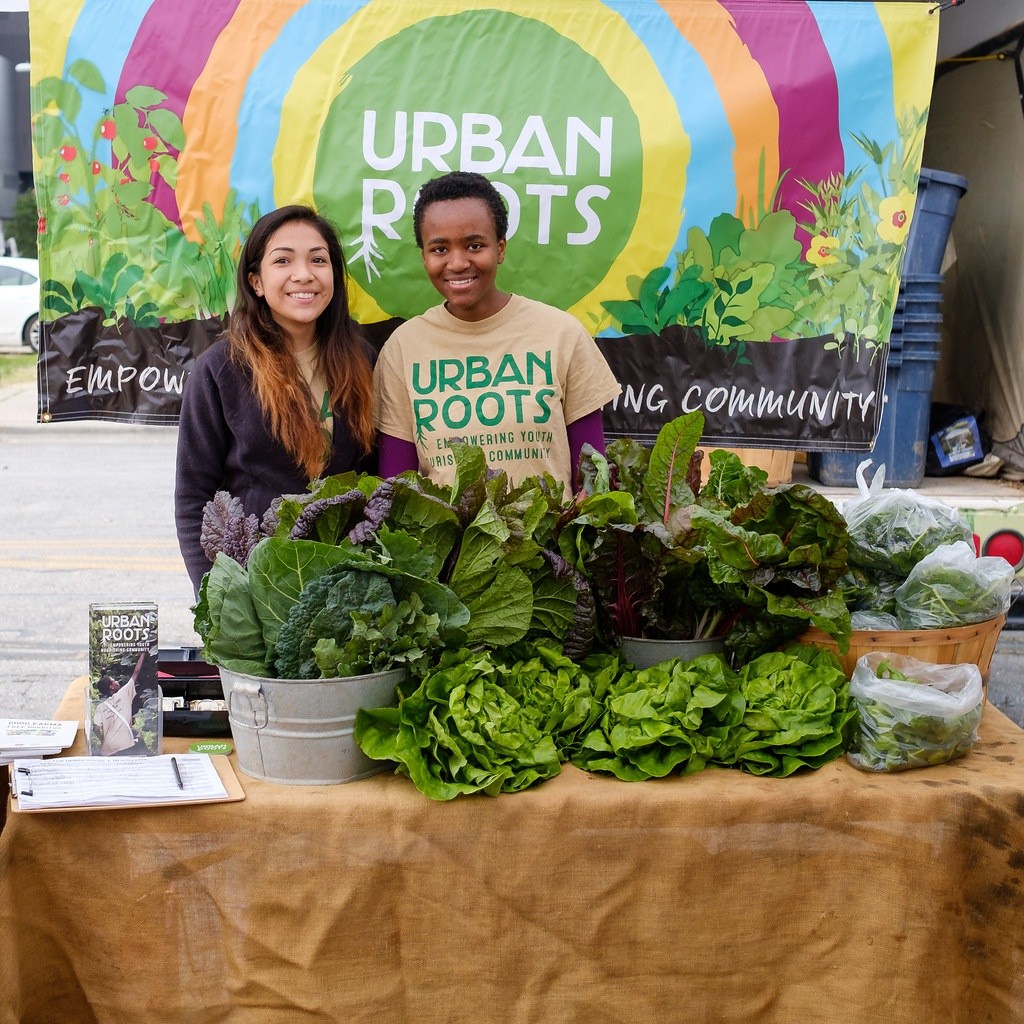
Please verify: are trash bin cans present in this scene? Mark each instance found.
[807,166,970,489]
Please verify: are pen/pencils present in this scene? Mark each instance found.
[171,757,183,790]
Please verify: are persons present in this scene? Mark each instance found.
[94,652,146,756]
[174,204,378,605]
[373,171,623,505]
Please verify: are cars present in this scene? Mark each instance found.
[0,256,42,353]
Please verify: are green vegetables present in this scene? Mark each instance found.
[842,488,1011,634]
[189,412,852,681]
[351,636,981,803]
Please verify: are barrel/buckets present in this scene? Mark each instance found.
[217,661,407,786]
[616,636,740,674]
[801,579,1024,711]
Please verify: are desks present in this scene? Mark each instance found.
[0,675,1024,1024]
